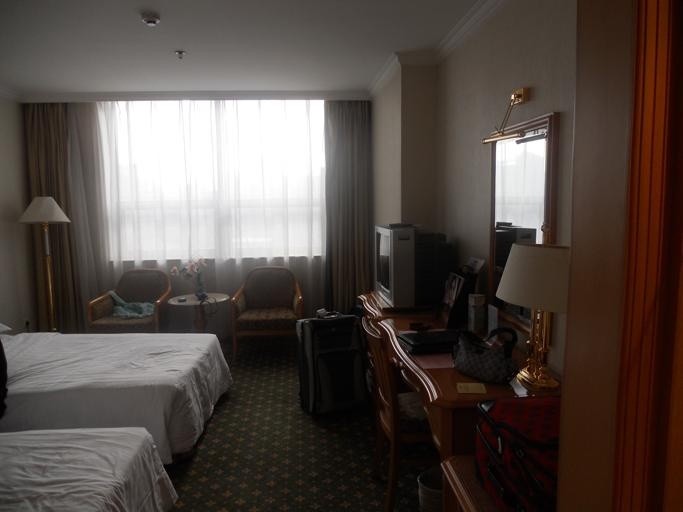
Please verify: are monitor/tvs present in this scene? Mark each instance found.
[493,226,536,266]
[373,224,451,309]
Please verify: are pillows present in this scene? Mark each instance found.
[1,323,13,335]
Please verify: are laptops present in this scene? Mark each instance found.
[397,331,456,354]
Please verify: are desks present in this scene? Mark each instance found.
[166,293,230,333]
[440,456,492,512]
[357,292,561,463]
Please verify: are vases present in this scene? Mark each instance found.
[192,276,207,301]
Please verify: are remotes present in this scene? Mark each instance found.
[388,223,412,228]
[497,222,512,226]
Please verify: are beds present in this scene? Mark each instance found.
[1,426,182,512]
[1,325,235,466]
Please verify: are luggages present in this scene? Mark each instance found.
[295,309,369,415]
[473,398,560,512]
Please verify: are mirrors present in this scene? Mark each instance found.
[481,110,558,349]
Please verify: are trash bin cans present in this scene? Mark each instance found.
[417,472,442,512]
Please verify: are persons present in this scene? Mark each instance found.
[443,275,458,306]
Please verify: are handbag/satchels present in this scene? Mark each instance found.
[454,328,517,386]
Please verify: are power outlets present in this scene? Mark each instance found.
[22,318,30,330]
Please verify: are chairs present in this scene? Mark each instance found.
[360,317,430,511]
[230,265,305,366]
[86,268,172,332]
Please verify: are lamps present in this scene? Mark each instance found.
[492,242,571,396]
[17,195,72,332]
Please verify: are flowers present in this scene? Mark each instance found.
[170,256,210,294]
